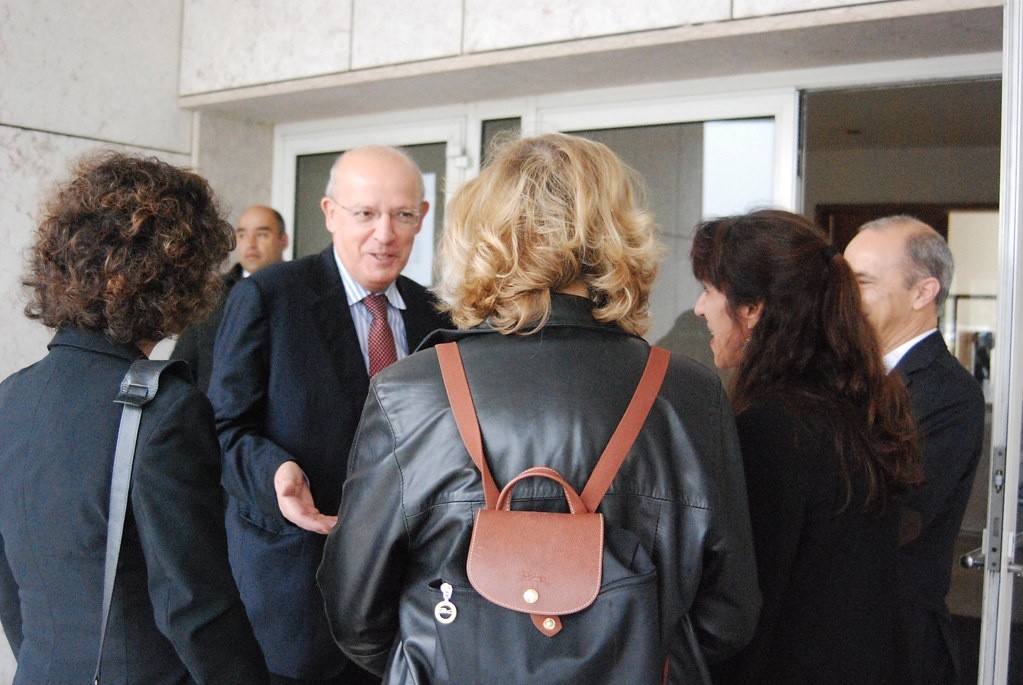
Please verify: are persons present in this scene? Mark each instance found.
[840,216,986,685]
[0,154,264,685]
[209,145,457,685]
[168,205,290,395]
[688,212,921,685]
[317,128,763,685]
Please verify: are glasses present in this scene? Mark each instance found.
[330,198,421,230]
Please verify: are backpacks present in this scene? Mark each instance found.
[426,342,672,685]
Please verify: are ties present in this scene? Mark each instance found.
[360,294,396,377]
